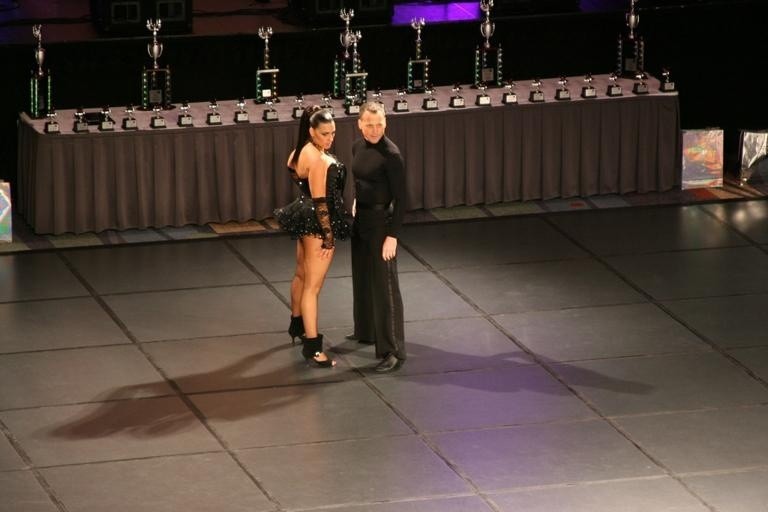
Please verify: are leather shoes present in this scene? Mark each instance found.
[374,353,403,374]
[344,331,358,341]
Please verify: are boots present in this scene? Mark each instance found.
[301,333,337,369]
[288,314,305,344]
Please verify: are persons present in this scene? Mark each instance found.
[345,100,407,373]
[273,102,348,368]
